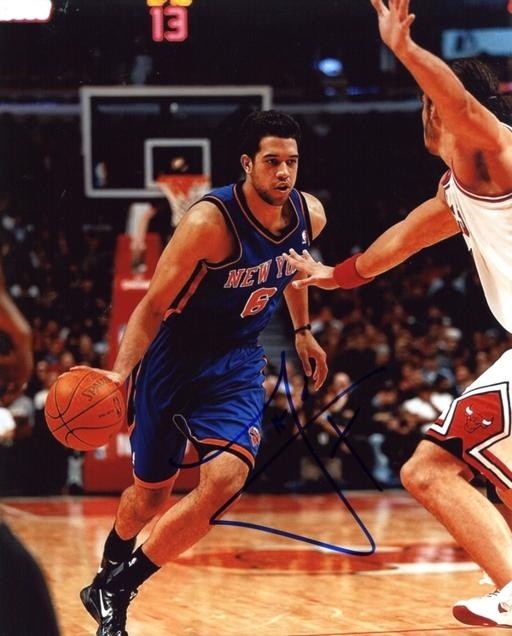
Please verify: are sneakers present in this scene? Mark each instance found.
[452,580,510,628]
[80,584,131,635]
[91,557,121,585]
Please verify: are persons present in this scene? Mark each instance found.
[1,291,66,636]
[278,3,512,634]
[0,202,512,496]
[65,111,334,636]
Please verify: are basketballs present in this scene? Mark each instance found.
[44,370,125,452]
